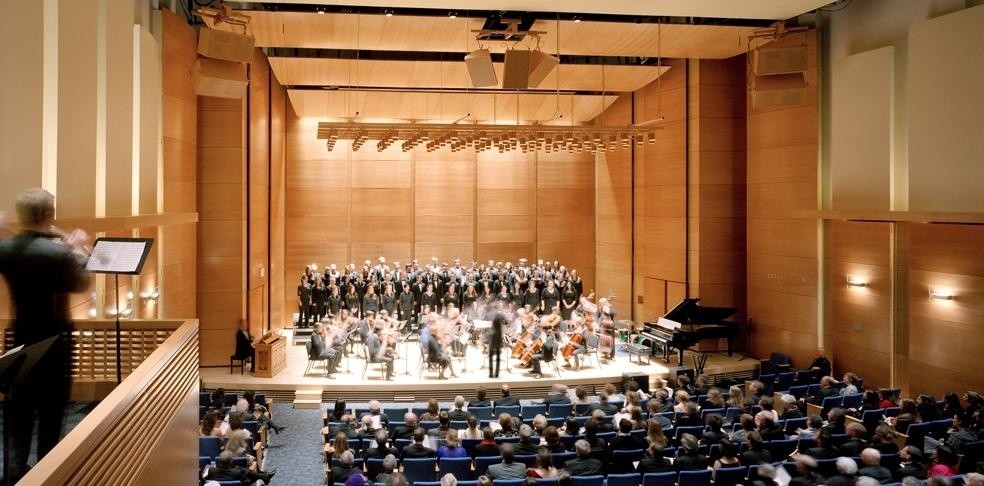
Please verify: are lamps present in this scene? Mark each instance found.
[317,123,664,153]
[465,17,561,89]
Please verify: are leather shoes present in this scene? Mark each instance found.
[276,424,288,433]
[264,470,276,484]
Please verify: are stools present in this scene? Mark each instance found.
[229,353,247,374]
[629,342,654,365]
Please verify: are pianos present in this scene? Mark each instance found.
[639,299,738,366]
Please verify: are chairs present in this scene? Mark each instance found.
[303,321,629,378]
[321,368,983,486]
[199,388,269,486]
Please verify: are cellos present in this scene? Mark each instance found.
[562,327,586,357]
[512,320,541,357]
[598,289,616,355]
[521,326,554,366]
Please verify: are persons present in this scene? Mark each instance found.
[199,386,286,486]
[234,317,255,373]
[1,188,95,486]
[321,346,983,485]
[297,257,617,379]
